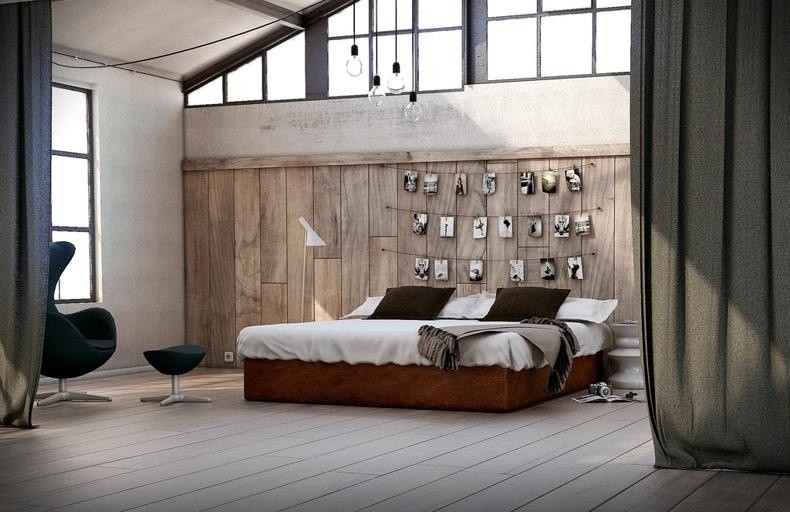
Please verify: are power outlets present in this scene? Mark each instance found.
[224,352,233,362]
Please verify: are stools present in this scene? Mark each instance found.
[139,343,212,408]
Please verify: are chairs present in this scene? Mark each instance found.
[31,240,117,407]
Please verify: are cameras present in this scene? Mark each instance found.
[588,382,613,398]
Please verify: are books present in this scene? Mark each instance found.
[572,394,641,403]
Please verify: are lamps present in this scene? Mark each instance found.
[344,0,426,125]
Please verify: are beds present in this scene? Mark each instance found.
[237,296,613,416]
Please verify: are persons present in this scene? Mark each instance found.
[475,220,484,235]
[404,173,410,189]
[415,261,428,275]
[414,214,419,225]
[486,177,493,193]
[566,169,581,186]
[555,217,569,232]
[456,178,463,195]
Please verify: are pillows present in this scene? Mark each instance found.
[336,285,620,326]
